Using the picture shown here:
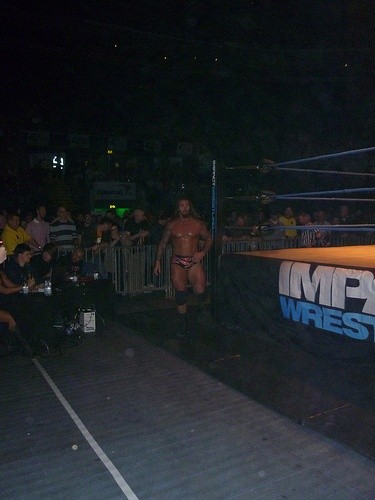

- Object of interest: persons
[154,197,213,340]
[195,198,375,290]
[0,199,173,358]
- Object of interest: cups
[22,284,29,294]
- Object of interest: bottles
[45,279,51,296]
